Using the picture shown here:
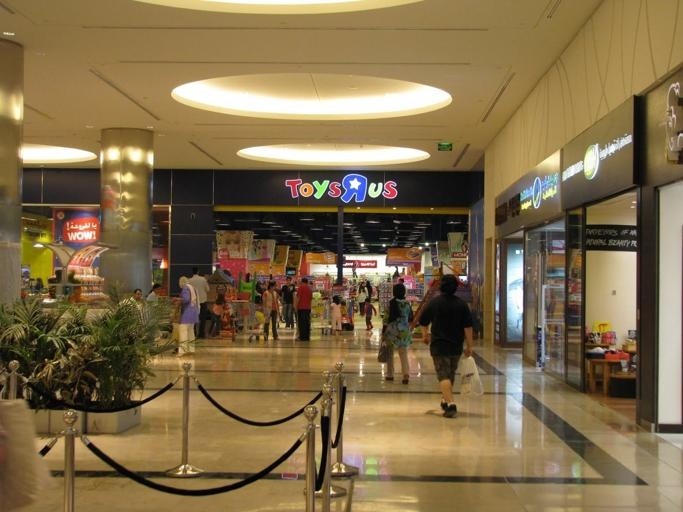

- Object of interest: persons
[330,279,372,335]
[257,277,312,340]
[364,299,377,330]
[130,272,210,356]
[379,282,413,385]
[418,274,474,418]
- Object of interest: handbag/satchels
[378,346,390,362]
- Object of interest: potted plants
[0,285,181,435]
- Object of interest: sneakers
[440,398,457,417]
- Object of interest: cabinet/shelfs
[584,336,636,395]
